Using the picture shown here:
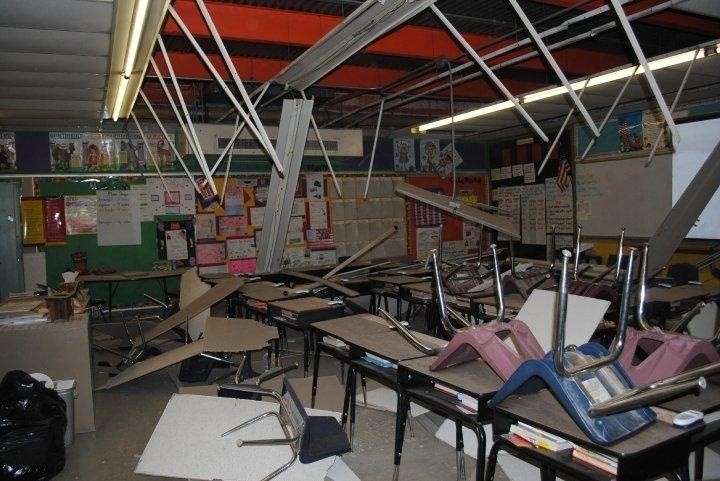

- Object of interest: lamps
[100,0,171,122]
[409,39,720,134]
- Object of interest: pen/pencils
[513,433,539,449]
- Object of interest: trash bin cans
[44,380,78,449]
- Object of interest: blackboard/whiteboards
[572,152,720,239]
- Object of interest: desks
[75,269,187,322]
[0,302,94,435]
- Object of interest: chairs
[95,221,720,481]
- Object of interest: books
[384,283,518,321]
[322,334,398,370]
[245,298,298,321]
[509,419,619,477]
[433,383,479,412]
